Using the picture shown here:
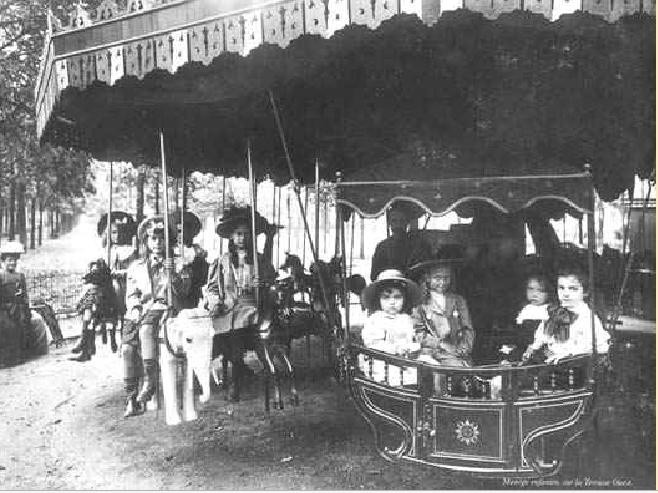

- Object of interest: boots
[73,321,93,361]
[122,375,143,417]
[136,359,157,402]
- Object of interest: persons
[69,209,284,417]
[369,206,429,275]
[0,241,34,363]
[409,248,476,370]
[498,271,557,368]
[357,269,441,393]
[490,269,612,400]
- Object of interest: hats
[407,240,468,270]
[1,242,26,253]
[98,202,270,249]
[362,270,420,310]
